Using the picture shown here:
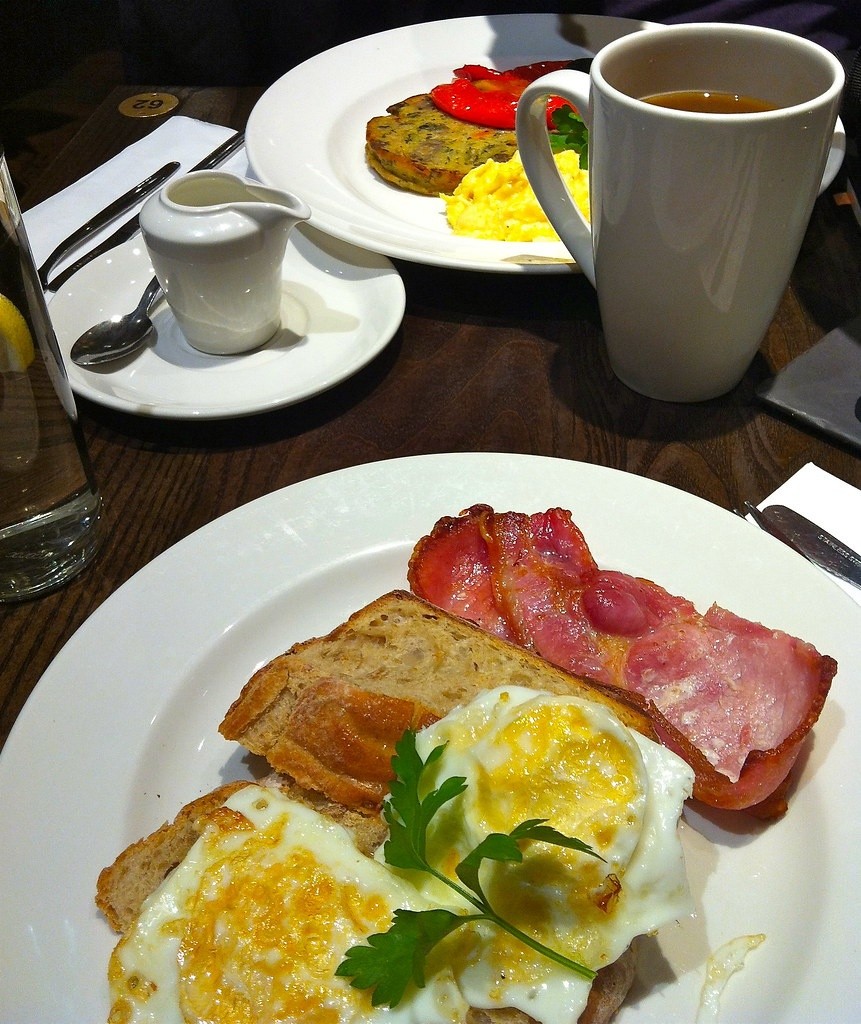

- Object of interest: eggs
[103,784,472,1024]
[374,683,697,1024]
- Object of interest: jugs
[139,169,312,356]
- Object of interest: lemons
[1,293,37,374]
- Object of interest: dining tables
[0,87,861,749]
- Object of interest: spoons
[70,274,161,365]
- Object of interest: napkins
[20,115,256,306]
[745,462,861,607]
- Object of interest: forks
[733,500,813,566]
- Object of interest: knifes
[764,505,861,590]
[47,128,245,294]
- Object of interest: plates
[22,116,406,420]
[0,451,861,1024]
[244,13,846,274]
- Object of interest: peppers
[428,62,581,129]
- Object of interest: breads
[217,587,657,844]
[96,770,640,1024]
[364,95,525,194]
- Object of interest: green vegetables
[543,102,589,171]
[333,728,610,1009]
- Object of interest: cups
[515,21,847,402]
[0,151,114,605]
